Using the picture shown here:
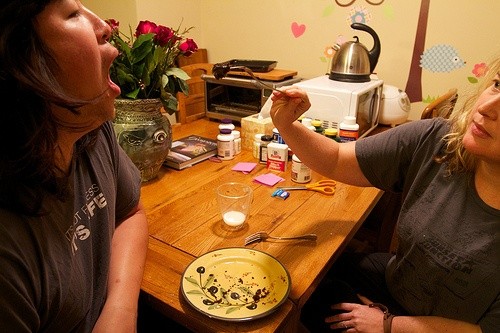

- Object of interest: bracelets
[368,303,394,333]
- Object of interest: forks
[245,230,317,246]
[230,66,287,96]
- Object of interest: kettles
[329,20,381,79]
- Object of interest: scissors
[276,180,337,195]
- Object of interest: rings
[343,321,347,328]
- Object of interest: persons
[0,0,147,333]
[269,56,500,333]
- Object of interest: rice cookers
[377,84,411,128]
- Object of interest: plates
[181,245,292,321]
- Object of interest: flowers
[104,19,198,116]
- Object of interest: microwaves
[291,74,384,138]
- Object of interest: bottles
[297,113,359,143]
[290,154,312,183]
[253,133,273,164]
[218,119,235,131]
[216,129,235,160]
[232,130,242,155]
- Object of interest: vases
[114,98,173,183]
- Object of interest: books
[162,134,218,171]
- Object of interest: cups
[272,128,285,145]
[216,182,253,232]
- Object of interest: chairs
[421,88,458,119]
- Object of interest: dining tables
[140,120,386,333]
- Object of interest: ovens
[199,74,303,122]
